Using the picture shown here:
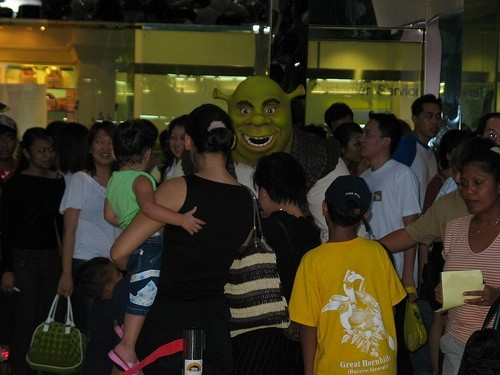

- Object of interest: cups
[182,329,203,375]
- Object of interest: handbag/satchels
[402,286,427,353]
[25,292,88,372]
[223,185,290,338]
[454,287,500,375]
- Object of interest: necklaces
[475,215,500,234]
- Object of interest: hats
[326,175,372,220]
[0,114,17,134]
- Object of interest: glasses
[486,132,500,140]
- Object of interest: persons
[0,94,500,375]
[212,77,330,193]
[104,120,206,375]
[289,175,407,375]
[110,104,258,375]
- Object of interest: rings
[481,298,483,302]
